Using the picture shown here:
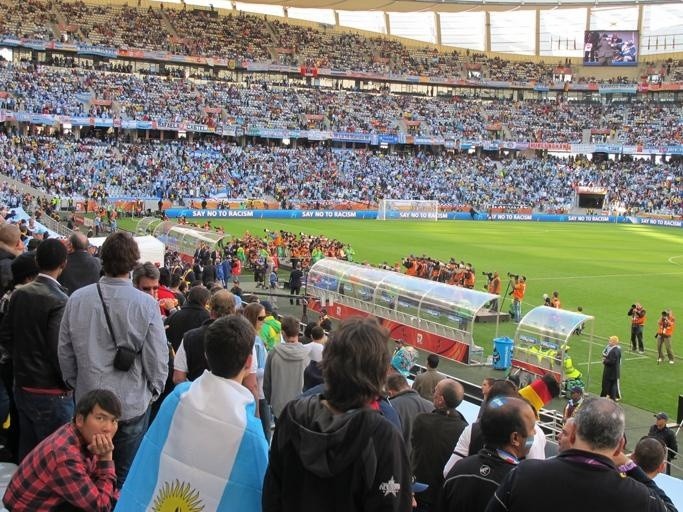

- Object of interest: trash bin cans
[492,336,514,370]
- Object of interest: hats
[395,339,403,344]
[568,386,582,394]
[654,413,667,419]
[411,472,429,493]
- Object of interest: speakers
[677,395,683,427]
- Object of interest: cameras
[632,304,636,308]
[662,311,669,317]
[507,272,518,280]
[482,272,492,281]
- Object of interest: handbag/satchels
[114,347,135,371]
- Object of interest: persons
[1,204,683,512]
[0,0,683,221]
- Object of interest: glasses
[258,317,266,321]
[143,286,158,291]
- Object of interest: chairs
[508,344,584,398]
[302,284,484,366]
[139,230,199,264]
